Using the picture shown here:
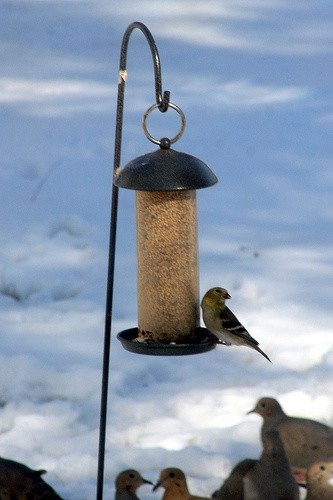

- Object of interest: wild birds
[242,430,299,500]
[304,461,333,500]
[114,470,153,500]
[200,287,272,362]
[13,470,50,500]
[210,458,260,500]
[153,468,210,500]
[244,397,333,476]
